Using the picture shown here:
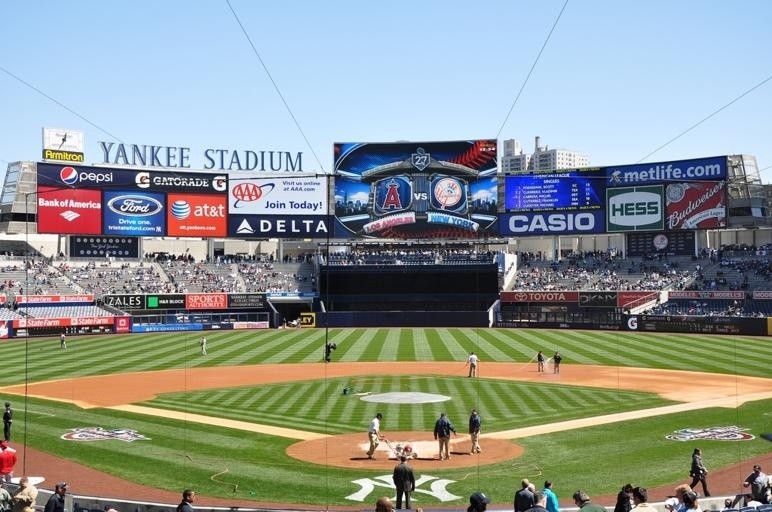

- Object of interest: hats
[572,490,589,501]
[54,481,71,490]
[751,463,762,472]
[376,412,383,418]
[683,490,701,500]
[469,491,492,503]
[1,442,8,449]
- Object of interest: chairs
[706,502,772,512]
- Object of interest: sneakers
[439,455,452,462]
[468,448,483,456]
[367,451,377,461]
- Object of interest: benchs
[1,256,132,324]
[316,249,500,270]
[133,261,315,293]
[639,250,770,317]
[506,255,641,300]
